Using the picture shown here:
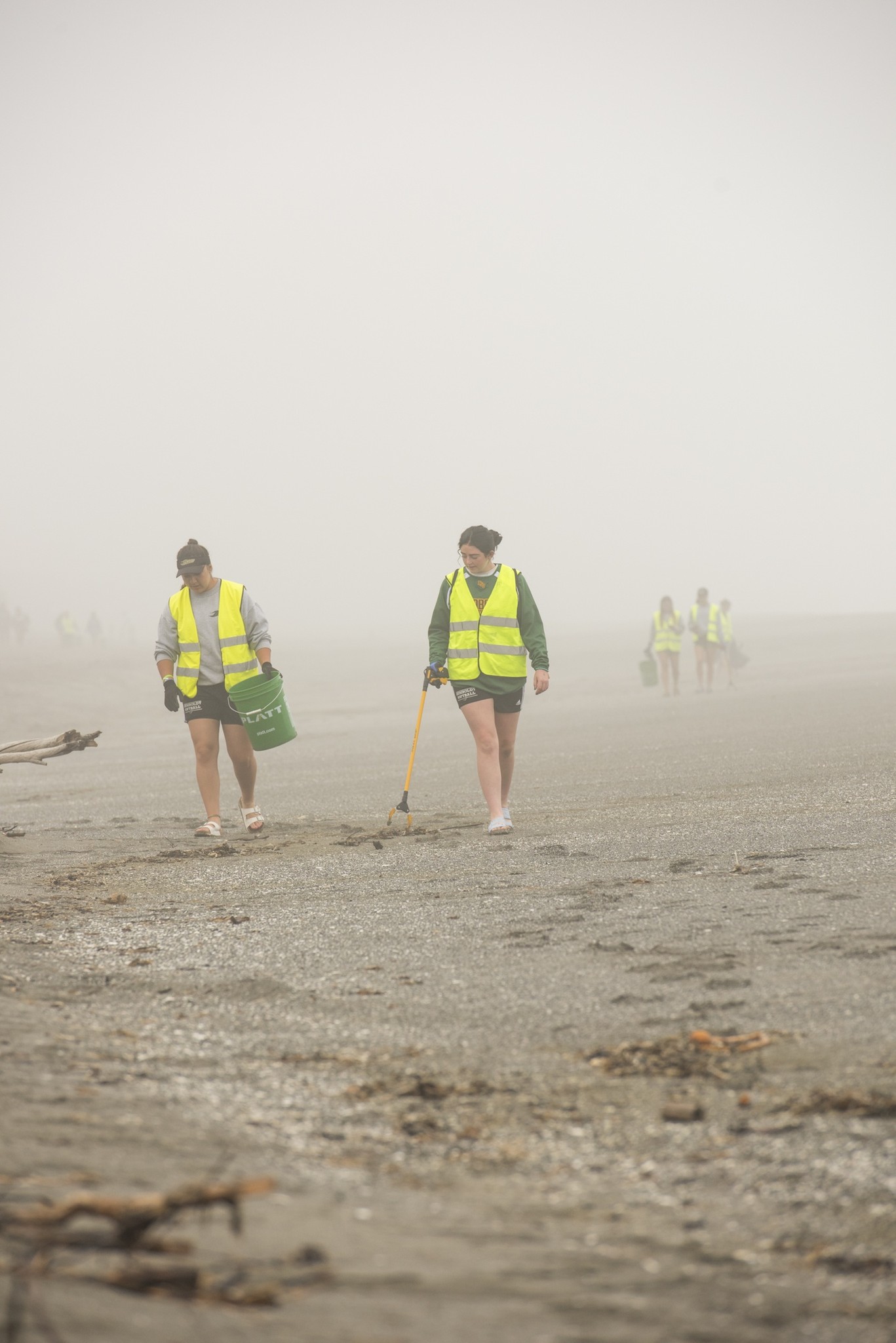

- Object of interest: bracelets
[162,674,173,681]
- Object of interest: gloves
[430,661,442,689]
[261,661,284,679]
[164,680,184,713]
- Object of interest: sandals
[238,798,264,834]
[194,822,223,836]
[488,818,508,835]
[503,808,515,834]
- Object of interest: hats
[176,544,210,579]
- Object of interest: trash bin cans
[639,661,658,686]
[228,671,297,751]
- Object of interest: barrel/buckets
[227,670,299,754]
[638,660,658,687]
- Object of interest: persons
[427,526,551,835]
[690,587,739,690]
[154,538,285,838]
[643,595,686,697]
